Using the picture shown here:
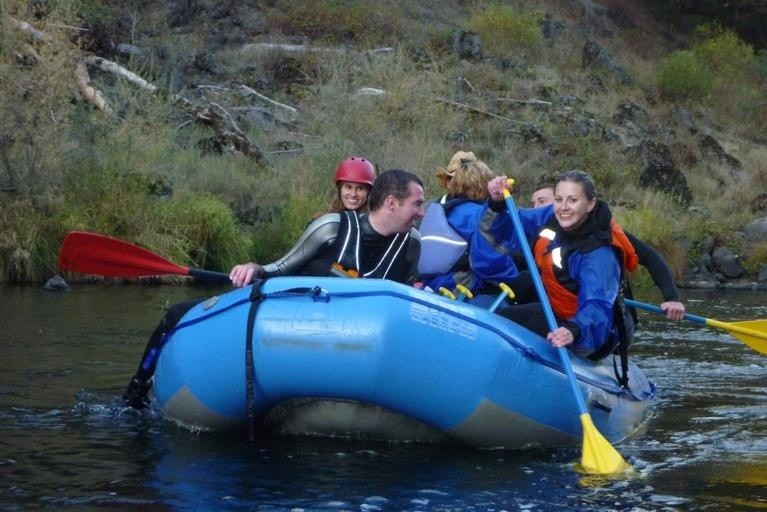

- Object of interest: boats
[152,276,657,451]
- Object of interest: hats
[437,151,477,188]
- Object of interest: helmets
[336,157,375,186]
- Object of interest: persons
[468,170,637,361]
[413,161,518,305]
[530,184,685,321]
[439,151,479,194]
[227,167,427,294]
[306,156,377,230]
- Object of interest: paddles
[623,296,766,356]
[57,232,256,283]
[498,177,632,478]
